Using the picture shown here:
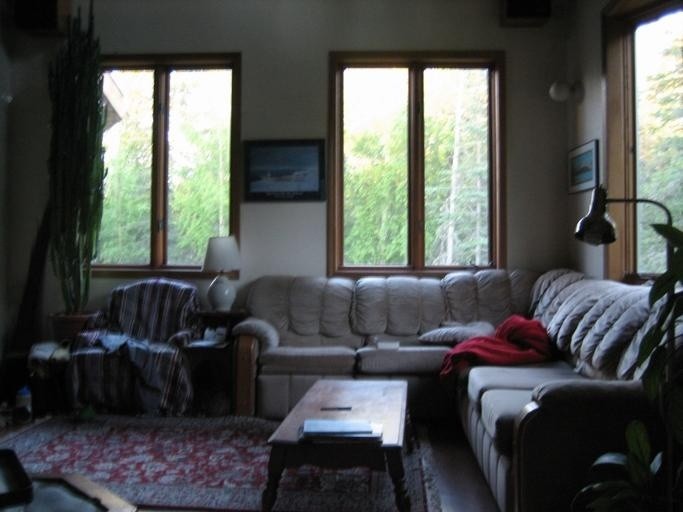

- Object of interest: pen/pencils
[319,407,351,411]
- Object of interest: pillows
[418,322,494,344]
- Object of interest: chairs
[74,278,200,418]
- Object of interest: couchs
[236,266,683,511]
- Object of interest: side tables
[186,308,243,415]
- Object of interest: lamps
[574,189,682,298]
[202,235,241,312]
[548,79,583,103]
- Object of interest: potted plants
[47,1,105,344]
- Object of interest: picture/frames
[244,138,326,201]
[567,139,600,194]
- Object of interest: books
[294,417,385,449]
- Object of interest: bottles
[14,384,33,424]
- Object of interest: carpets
[1,413,442,512]
